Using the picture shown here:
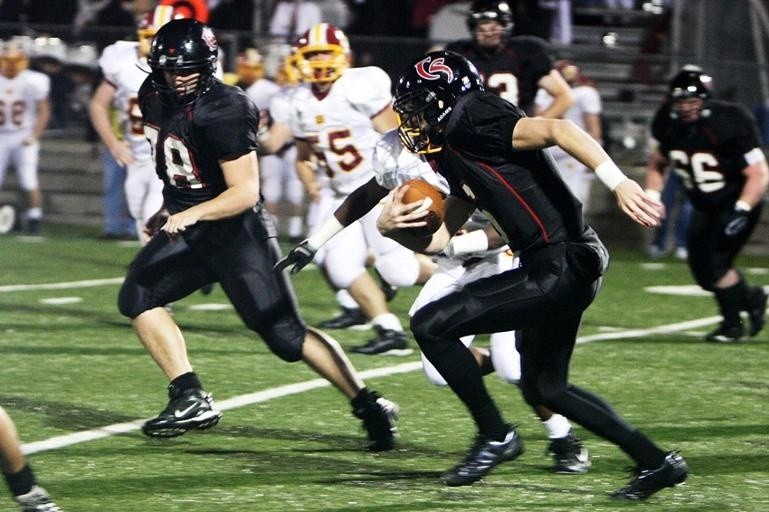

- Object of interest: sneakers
[613,451,688,503]
[318,306,368,331]
[350,328,413,355]
[546,426,591,475]
[438,425,522,486]
[707,322,744,342]
[749,285,768,338]
[15,483,62,511]
[352,392,400,450]
[143,388,220,439]
[25,220,38,235]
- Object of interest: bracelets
[645,188,661,201]
[734,201,752,212]
[594,159,626,191]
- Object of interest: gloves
[723,201,752,236]
[269,240,317,276]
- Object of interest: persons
[646,64,704,261]
[271,127,591,477]
[376,50,690,503]
[644,63,768,344]
[1,0,605,358]
[113,17,403,451]
[0,402,64,512]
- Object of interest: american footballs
[394,178,445,235]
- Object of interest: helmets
[391,50,484,154]
[669,63,714,120]
[467,1,513,59]
[285,23,350,84]
[0,45,28,76]
[234,47,265,80]
[136,7,216,108]
